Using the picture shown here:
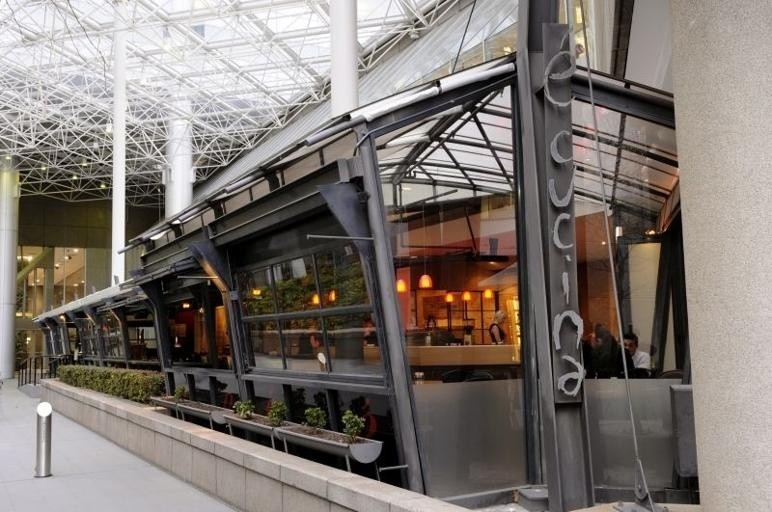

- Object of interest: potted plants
[150,385,384,464]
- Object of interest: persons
[590,327,636,379]
[488,309,508,345]
[296,319,322,360]
[363,319,379,347]
[623,332,651,371]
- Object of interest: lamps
[394,201,495,302]
